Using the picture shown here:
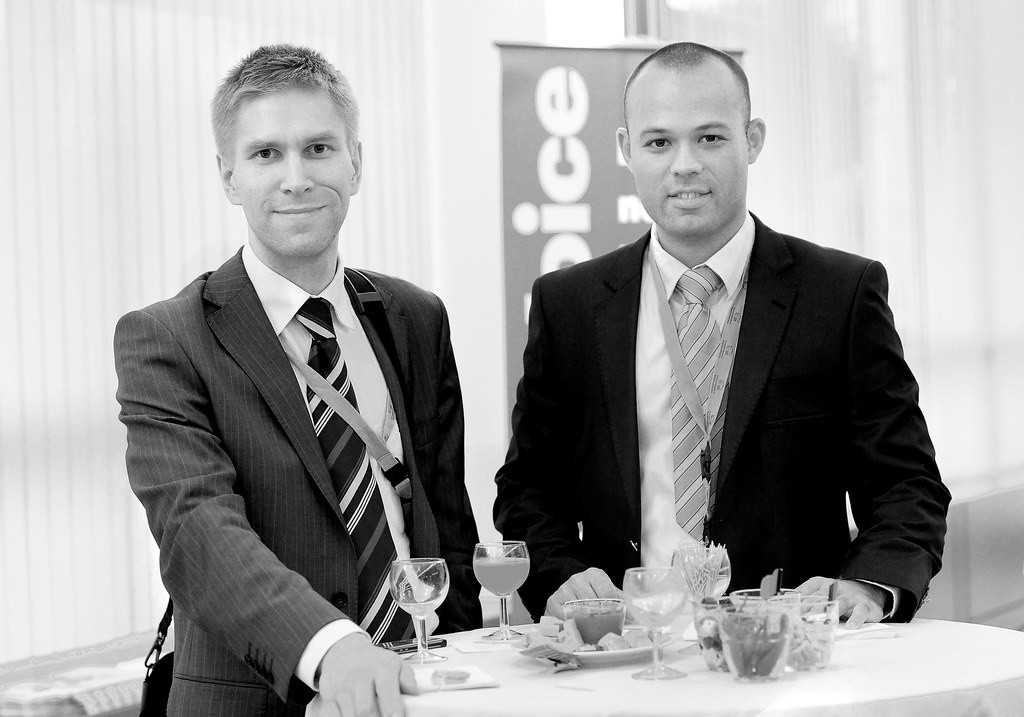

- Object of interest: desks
[305,617,1024,716]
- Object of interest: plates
[546,632,677,668]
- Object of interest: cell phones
[376,636,447,654]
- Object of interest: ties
[294,297,418,649]
[673,265,725,547]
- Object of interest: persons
[114,42,485,717]
[492,41,953,627]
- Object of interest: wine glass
[474,540,531,642]
[390,557,451,664]
[623,567,689,680]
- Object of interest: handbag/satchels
[140,650,176,717]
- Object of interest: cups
[563,598,627,645]
[671,544,840,683]
